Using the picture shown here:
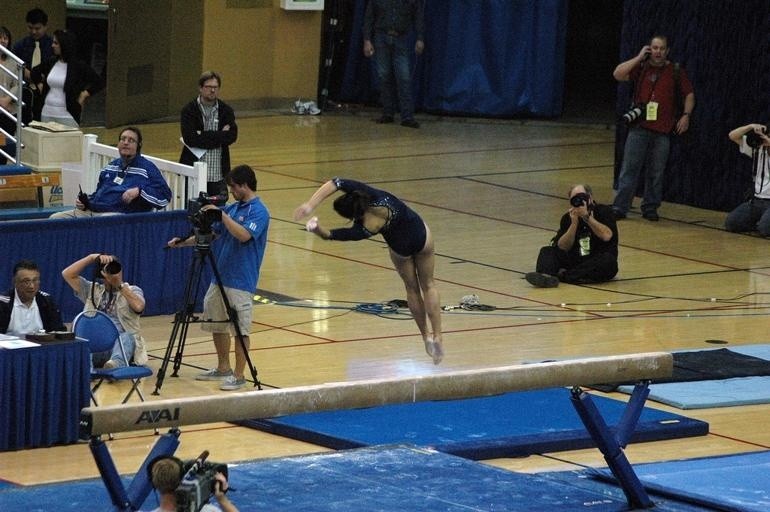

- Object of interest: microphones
[126,147,140,158]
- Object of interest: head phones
[146,454,185,489]
[138,137,142,147]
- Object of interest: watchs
[584,212,591,221]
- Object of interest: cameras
[571,192,589,208]
[623,104,646,125]
[747,131,764,146]
[94,259,122,279]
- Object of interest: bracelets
[683,111,695,119]
[117,282,129,290]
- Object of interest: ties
[29,41,41,68]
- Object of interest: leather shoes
[400,119,419,128]
[614,209,627,221]
[376,115,394,124]
[642,210,659,221]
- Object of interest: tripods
[149,248,264,394]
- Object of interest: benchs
[0,172,77,220]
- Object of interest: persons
[606,33,695,222]
[294,178,447,365]
[49,130,170,219]
[358,1,433,129]
[180,73,237,202]
[146,455,246,512]
[0,11,101,141]
[525,183,620,290]
[1,264,68,338]
[724,122,770,237]
[168,165,270,395]
[62,255,148,379]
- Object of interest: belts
[374,27,408,37]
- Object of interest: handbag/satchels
[675,62,705,137]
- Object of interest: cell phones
[645,52,651,59]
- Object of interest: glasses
[120,136,138,145]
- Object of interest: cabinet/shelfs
[20,125,83,208]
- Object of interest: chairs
[72,309,159,440]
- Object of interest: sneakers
[103,359,117,382]
[220,375,247,391]
[558,268,568,282]
[289,101,307,115]
[526,272,559,287]
[305,101,321,116]
[196,368,233,381]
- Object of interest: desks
[0,332,90,451]
